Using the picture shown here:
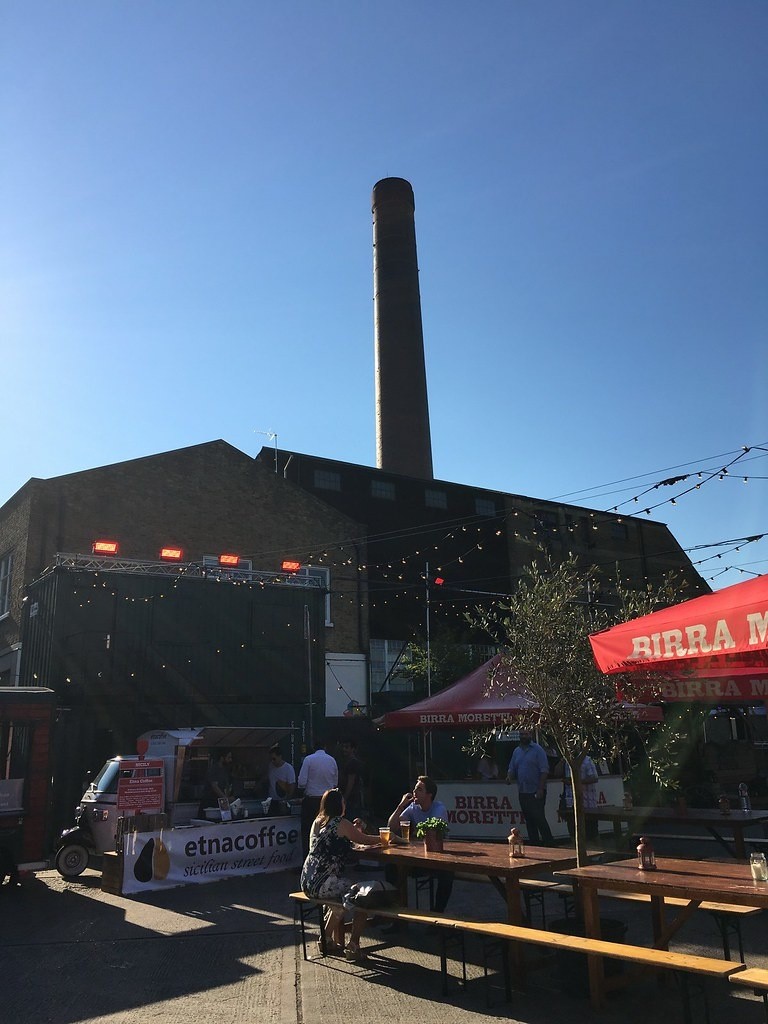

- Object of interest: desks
[359,835,607,992]
[552,855,768,1018]
[556,802,768,866]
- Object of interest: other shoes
[378,918,408,933]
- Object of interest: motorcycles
[55,727,307,879]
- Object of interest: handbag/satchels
[558,791,566,811]
[341,879,401,910]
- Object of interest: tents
[385,651,664,779]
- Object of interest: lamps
[281,560,302,575]
[93,539,119,555]
[159,546,184,560]
[219,554,241,568]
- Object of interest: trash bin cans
[547,916,629,985]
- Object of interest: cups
[379,827,390,846]
[400,821,410,840]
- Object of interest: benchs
[289,834,768,1015]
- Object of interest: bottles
[739,780,751,813]
[750,852,767,881]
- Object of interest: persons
[267,747,296,814]
[477,755,498,780]
[298,738,339,859]
[385,775,454,913]
[336,745,361,818]
[506,727,554,847]
[301,789,384,958]
[198,748,232,818]
[555,738,599,847]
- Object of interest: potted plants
[415,818,450,853]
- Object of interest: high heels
[318,936,345,954]
[344,941,370,960]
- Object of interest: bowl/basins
[204,809,221,818]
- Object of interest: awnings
[588,573,768,673]
[615,668,768,704]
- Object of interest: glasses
[271,758,276,761]
[335,787,341,794]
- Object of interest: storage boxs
[100,850,122,895]
